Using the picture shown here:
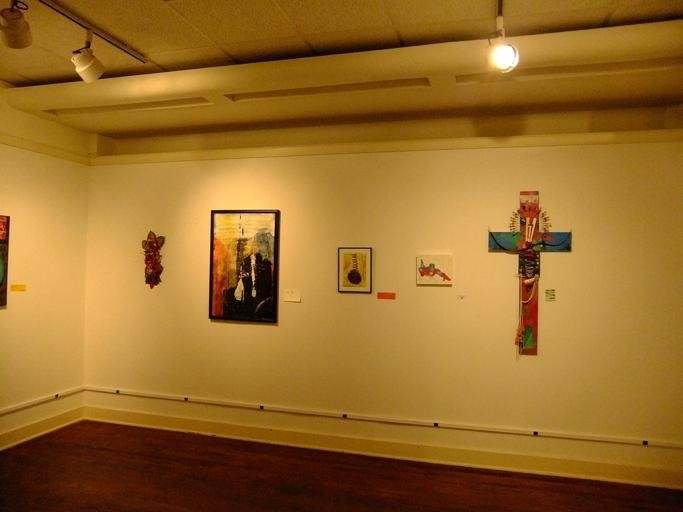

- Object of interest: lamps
[0,0,107,86]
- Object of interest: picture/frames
[206,208,280,326]
[336,247,372,295]
